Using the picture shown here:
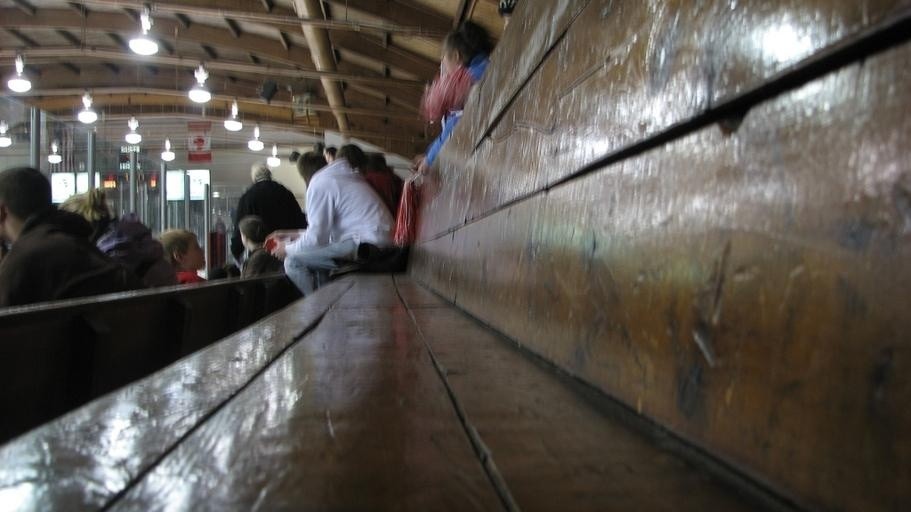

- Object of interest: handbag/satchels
[354,243,406,273]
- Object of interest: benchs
[0,0,909,510]
[0,275,299,444]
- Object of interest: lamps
[77,92,99,126]
[269,143,279,168]
[186,62,213,106]
[4,55,35,92]
[159,136,178,162]
[47,143,62,164]
[123,115,143,146]
[125,3,162,58]
[2,120,12,150]
[245,122,265,151]
[222,98,245,132]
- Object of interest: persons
[416,19,488,172]
[0,168,205,309]
[238,214,279,287]
[230,161,308,265]
[267,147,409,296]
